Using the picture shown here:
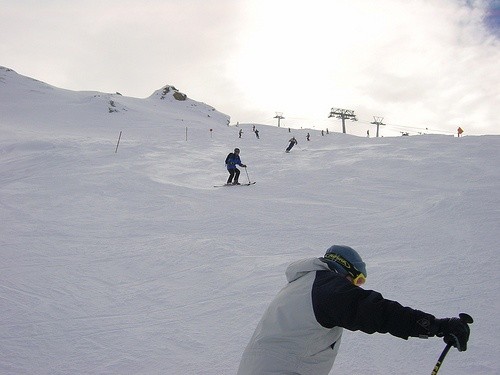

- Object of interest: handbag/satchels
[226,153,234,164]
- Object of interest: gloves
[436,318,470,352]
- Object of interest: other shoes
[227,182,232,184]
[234,181,238,183]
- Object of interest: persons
[252,125,255,131]
[305,133,311,141]
[457,127,463,137]
[236,243,471,375]
[239,129,244,138]
[326,128,329,134]
[288,128,291,132]
[367,129,369,137]
[320,130,325,136]
[284,136,298,153]
[225,147,249,185]
[255,129,260,139]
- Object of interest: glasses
[324,253,366,286]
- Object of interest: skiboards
[214,182,256,187]
[284,149,291,153]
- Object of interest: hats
[235,148,240,152]
[324,244,367,280]
